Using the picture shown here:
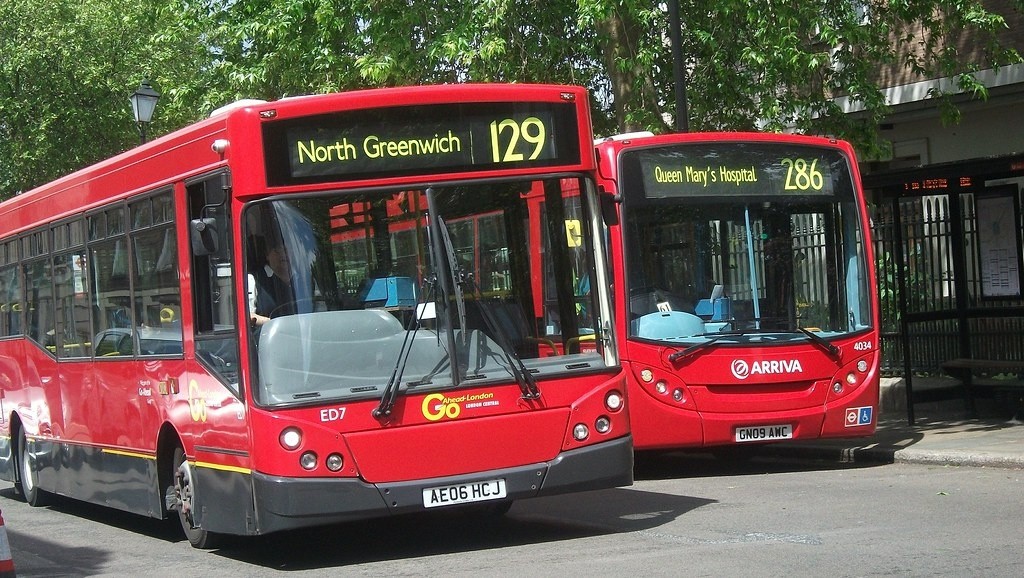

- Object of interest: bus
[0,82,634,551]
[326,130,881,471]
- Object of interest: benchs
[942,358,1024,418]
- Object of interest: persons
[247,234,327,328]
[577,244,639,325]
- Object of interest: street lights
[129,75,161,146]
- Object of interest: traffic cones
[0,509,17,578]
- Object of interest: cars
[92,324,240,403]
